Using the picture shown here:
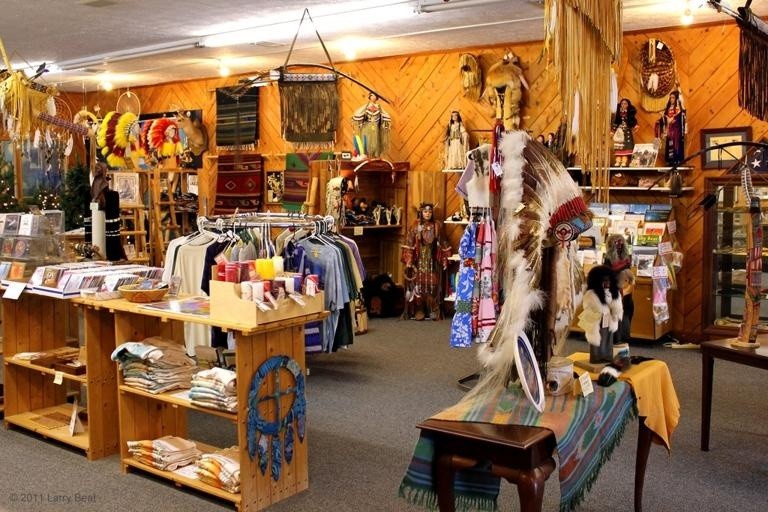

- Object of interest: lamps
[55,37,205,71]
[411,0,495,14]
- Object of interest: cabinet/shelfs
[103,294,331,512]
[701,175,768,337]
[442,167,695,339]
[0,285,120,460]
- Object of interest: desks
[416,352,666,512]
[700,334,767,452]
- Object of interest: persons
[610,99,640,167]
[351,92,392,160]
[444,111,469,168]
[603,234,634,344]
[545,133,554,148]
[655,91,685,167]
[536,135,546,146]
[577,267,623,364]
[401,204,452,321]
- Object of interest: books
[576,203,674,277]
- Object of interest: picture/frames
[700,126,752,170]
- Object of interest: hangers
[186,210,342,258]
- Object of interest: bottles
[240,272,319,302]
[92,208,107,260]
[84,217,92,242]
[544,356,574,397]
[211,256,284,283]
[613,342,631,370]
